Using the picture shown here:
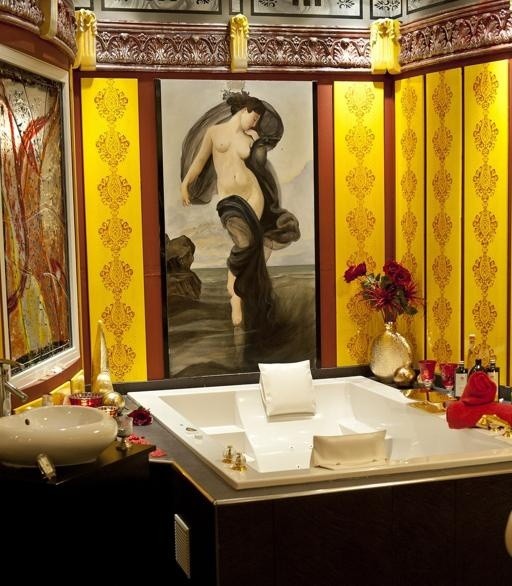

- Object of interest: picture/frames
[0,42,82,391]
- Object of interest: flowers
[343,258,425,322]
[126,406,152,426]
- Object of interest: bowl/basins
[68,391,118,417]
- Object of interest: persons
[181,92,299,365]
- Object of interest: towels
[446,400,512,429]
[461,371,497,405]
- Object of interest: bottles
[455,358,500,402]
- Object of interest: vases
[367,323,414,383]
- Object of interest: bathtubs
[127,376,512,490]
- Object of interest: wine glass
[418,360,458,403]
[116,417,135,451]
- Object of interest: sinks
[1,404,118,469]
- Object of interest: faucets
[0,359,27,417]
